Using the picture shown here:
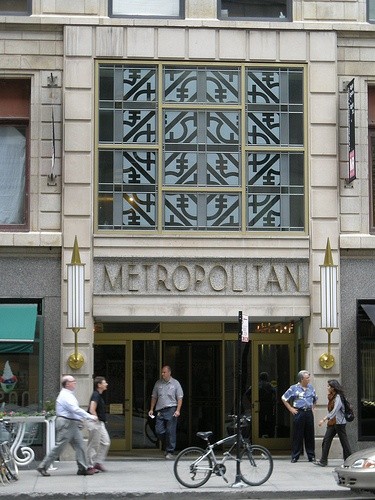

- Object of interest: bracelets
[323,419,325,421]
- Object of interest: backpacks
[340,399,354,422]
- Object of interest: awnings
[360,304,375,326]
[0,303,38,353]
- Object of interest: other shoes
[291,457,297,462]
[94,463,105,473]
[316,462,324,466]
[310,458,316,461]
[165,452,174,459]
[37,467,50,476]
[78,467,94,474]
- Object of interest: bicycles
[173,414,273,488]
[0,419,20,482]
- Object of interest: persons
[86,376,111,472]
[245,372,276,437]
[148,365,184,459]
[312,380,352,467]
[281,370,317,463]
[37,375,98,477]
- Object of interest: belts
[299,408,312,411]
